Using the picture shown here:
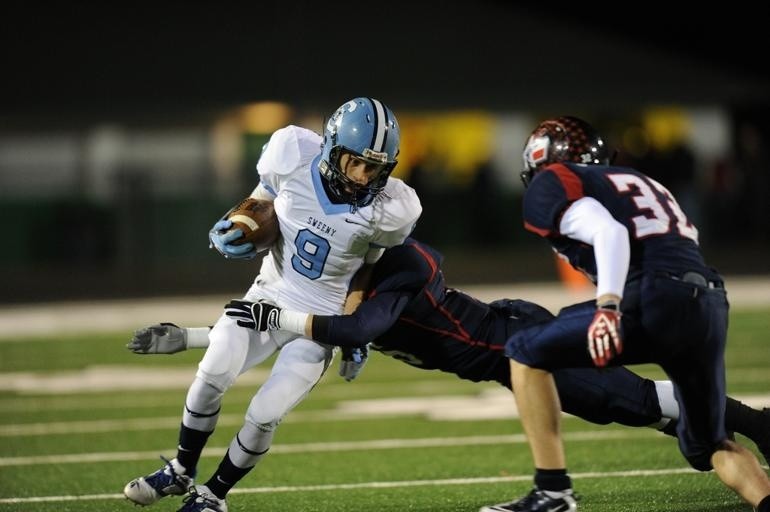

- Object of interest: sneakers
[123,458,195,507]
[478,486,578,512]
[176,486,227,512]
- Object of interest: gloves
[586,308,624,367]
[339,344,369,382]
[223,298,287,331]
[208,219,257,262]
[125,322,187,355]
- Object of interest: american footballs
[226,199,280,253]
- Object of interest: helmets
[322,96,401,165]
[520,116,611,179]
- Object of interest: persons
[122,99,423,512]
[125,233,769,468]
[475,116,770,512]
